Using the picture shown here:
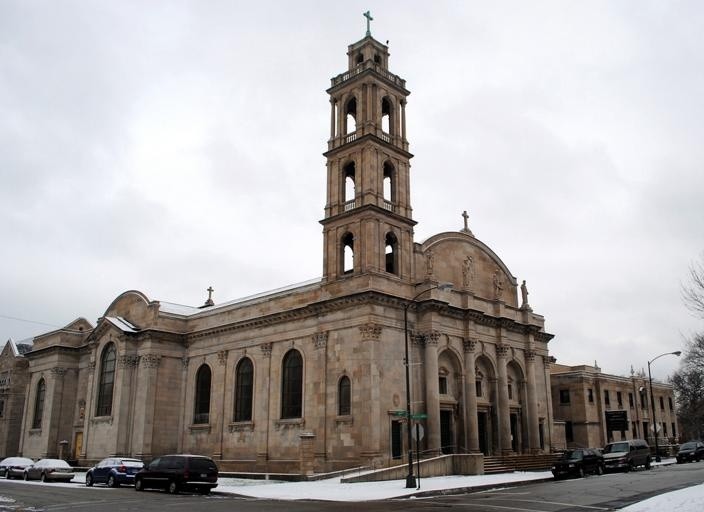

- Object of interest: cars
[552,448,605,480]
[676,442,704,464]
[86,458,145,486]
[0,457,34,480]
[23,459,74,481]
[134,456,218,494]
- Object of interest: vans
[602,439,651,473]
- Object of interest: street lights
[404,283,454,488]
[648,351,681,462]
